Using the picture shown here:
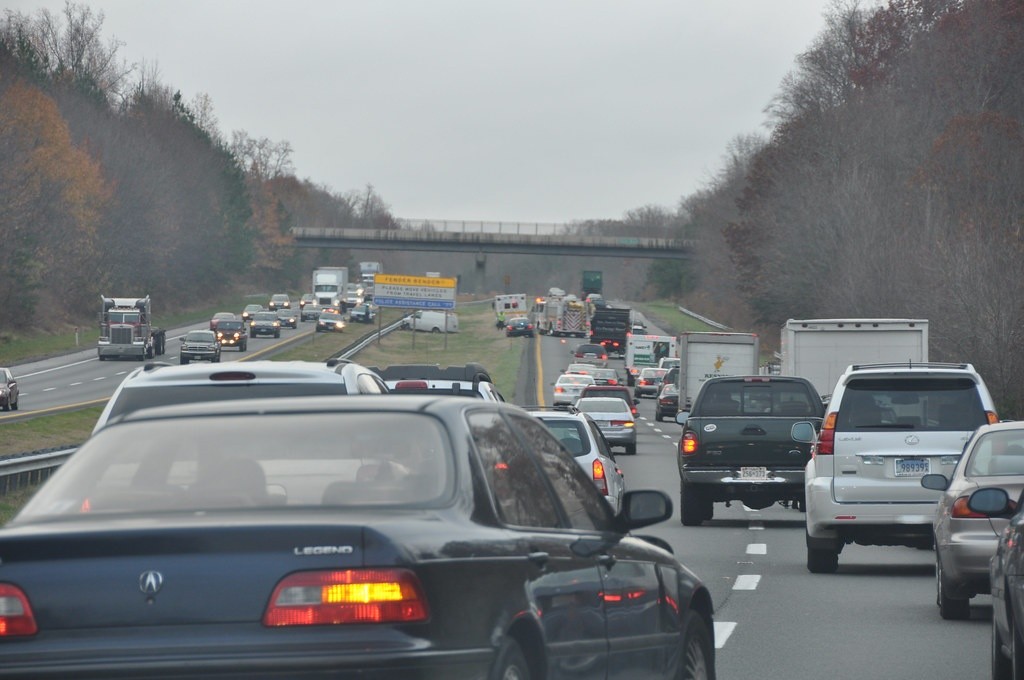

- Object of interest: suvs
[214,320,248,352]
[85,356,409,509]
[178,329,222,364]
[789,360,1000,573]
[674,374,829,526]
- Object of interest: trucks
[401,309,459,334]
[780,319,929,429]
[677,331,760,420]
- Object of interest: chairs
[770,400,809,416]
[701,399,741,417]
[987,453,1024,476]
[560,437,582,454]
[848,394,883,427]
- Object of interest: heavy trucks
[97,292,166,360]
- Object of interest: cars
[965,486,1024,680]
[917,419,1024,621]
[0,393,718,680]
[242,261,384,339]
[0,366,21,413]
[492,268,683,455]
[515,403,627,520]
[210,312,236,330]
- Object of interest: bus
[361,363,507,407]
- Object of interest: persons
[497,311,505,331]
